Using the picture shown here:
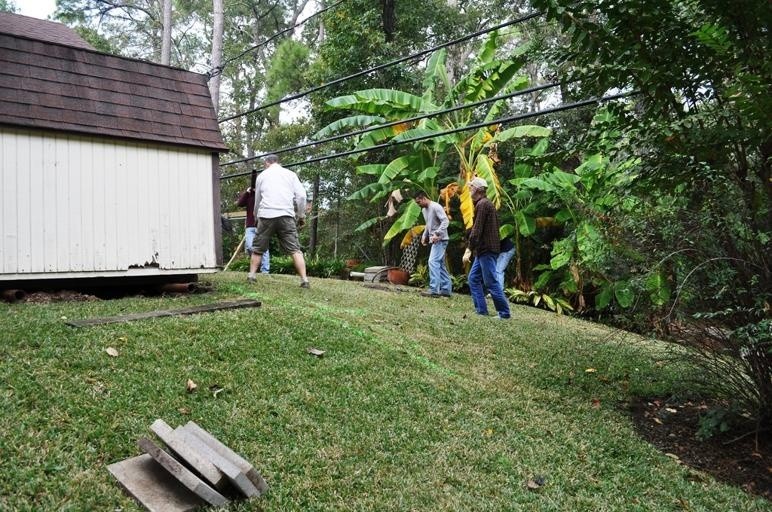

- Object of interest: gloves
[462,248,473,263]
[296,217,305,226]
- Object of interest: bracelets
[465,251,471,253]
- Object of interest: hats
[467,178,489,190]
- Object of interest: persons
[461,176,513,322]
[235,187,271,275]
[246,154,311,290]
[413,190,453,299]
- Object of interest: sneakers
[440,293,451,297]
[246,276,257,282]
[300,283,309,288]
[491,315,500,320]
[422,292,438,298]
